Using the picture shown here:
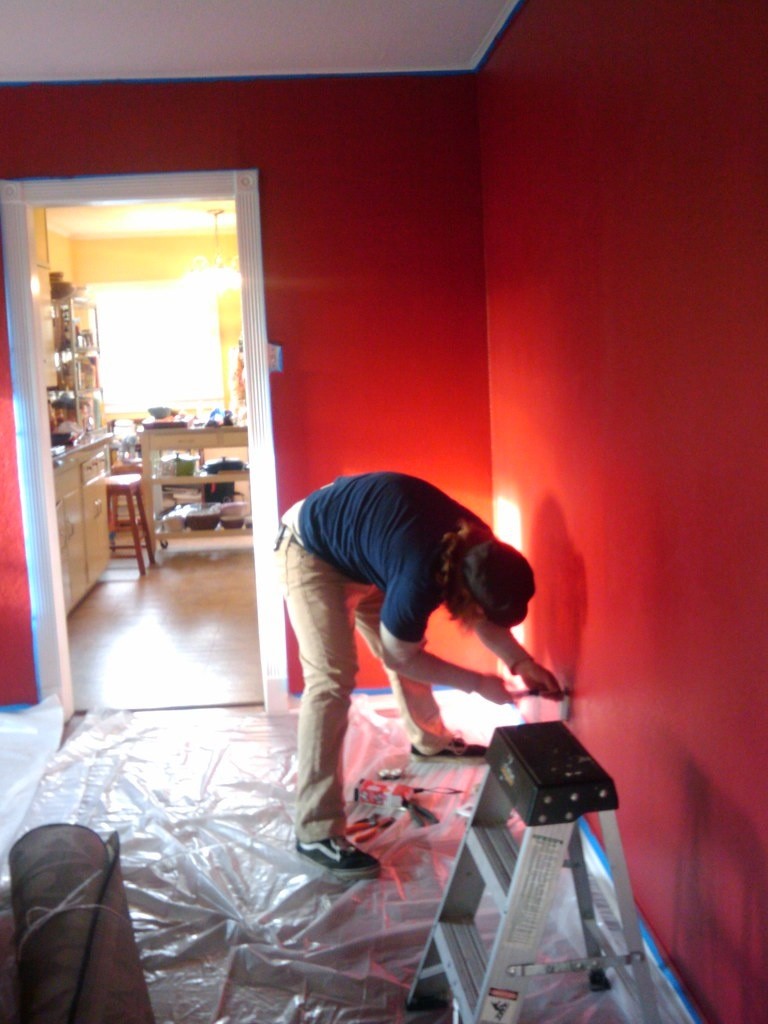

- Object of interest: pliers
[403,794,440,828]
[346,816,395,844]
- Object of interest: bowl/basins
[220,518,244,529]
[186,514,220,530]
[148,408,170,419]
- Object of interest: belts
[282,526,300,546]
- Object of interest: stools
[105,474,155,576]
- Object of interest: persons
[273,471,563,878]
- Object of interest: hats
[464,540,535,628]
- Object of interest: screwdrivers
[510,688,565,699]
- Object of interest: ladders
[403,720,659,1024]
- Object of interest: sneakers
[411,730,488,764]
[296,835,380,878]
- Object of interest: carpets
[9,824,156,1024]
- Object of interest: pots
[203,456,244,474]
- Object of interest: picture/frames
[33,207,52,269]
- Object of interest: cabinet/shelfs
[140,424,253,548]
[53,298,102,438]
[51,432,114,616]
